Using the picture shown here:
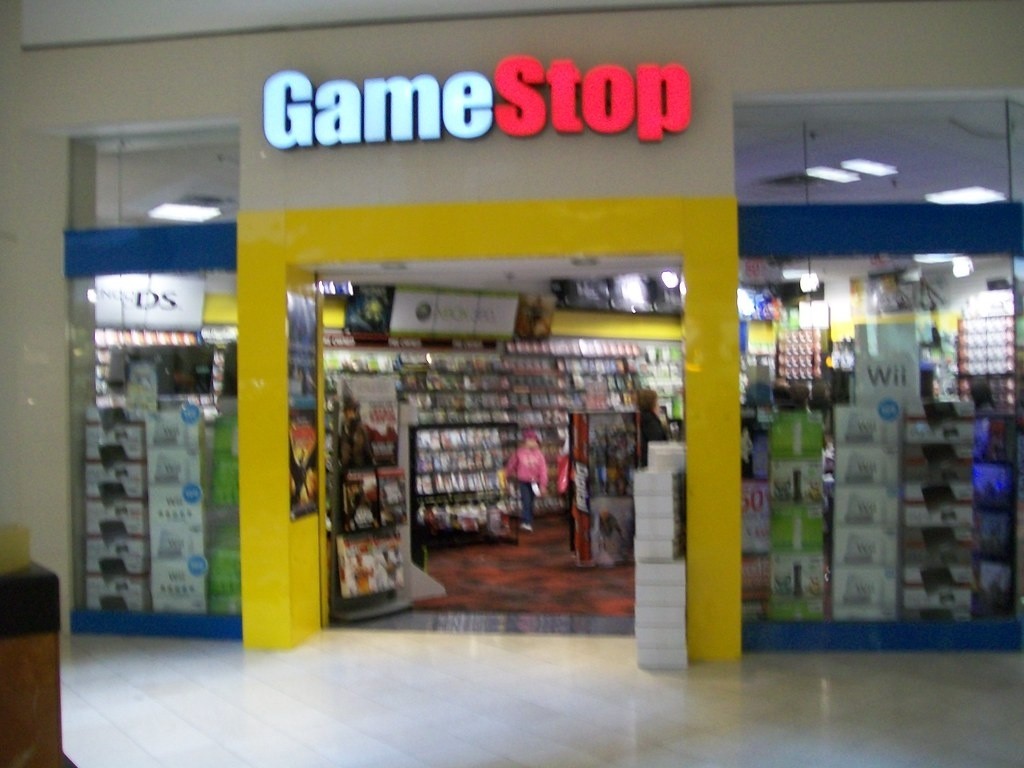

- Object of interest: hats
[523,430,537,442]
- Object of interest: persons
[507,431,548,532]
[343,539,402,598]
[638,390,668,443]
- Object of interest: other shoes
[519,524,533,534]
